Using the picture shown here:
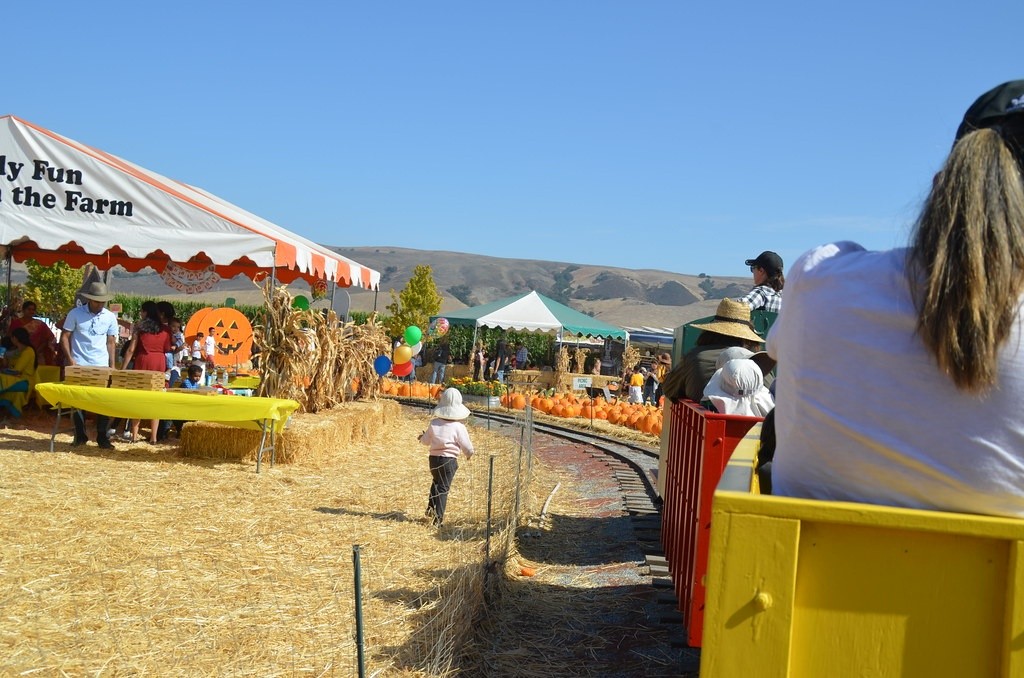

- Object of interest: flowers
[442,377,506,397]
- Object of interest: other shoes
[425,507,434,517]
[176,431,181,439]
[70,436,86,447]
[122,430,131,440]
[433,521,443,527]
[107,428,117,435]
[99,442,115,450]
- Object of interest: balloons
[373,318,450,376]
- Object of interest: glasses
[751,267,760,273]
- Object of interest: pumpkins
[498,389,663,436]
[382,380,443,399]
[223,363,264,377]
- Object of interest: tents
[0,115,381,339]
[430,290,630,354]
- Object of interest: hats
[658,352,672,364]
[434,388,470,420]
[640,367,647,373]
[745,251,783,276]
[953,79,1024,171]
[689,298,767,342]
[715,345,775,376]
[81,282,115,302]
[702,358,775,417]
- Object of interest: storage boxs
[64,365,166,392]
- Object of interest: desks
[512,369,540,391]
[36,381,300,475]
[175,373,261,389]
[37,365,60,405]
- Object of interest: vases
[462,393,500,407]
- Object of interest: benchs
[509,382,542,385]
[698,422,1024,678]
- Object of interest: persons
[622,352,672,408]
[664,251,786,417]
[756,78,1024,519]
[474,339,553,386]
[417,388,474,528]
[393,333,450,384]
[0,282,224,450]
